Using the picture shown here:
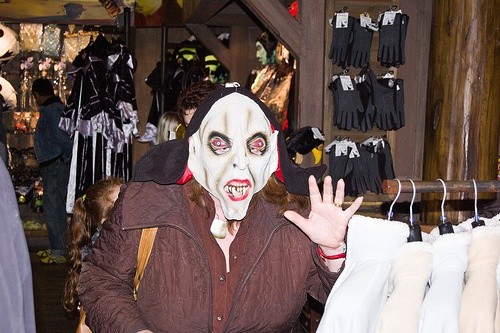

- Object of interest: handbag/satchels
[76,227,158,333]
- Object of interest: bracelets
[318,243,346,259]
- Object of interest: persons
[32,77,75,263]
[62,178,128,333]
[247,31,276,101]
[77,85,364,333]
[176,80,216,127]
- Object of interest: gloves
[325,5,410,197]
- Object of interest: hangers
[388,178,485,242]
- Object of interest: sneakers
[37,249,52,257]
[40,254,69,265]
